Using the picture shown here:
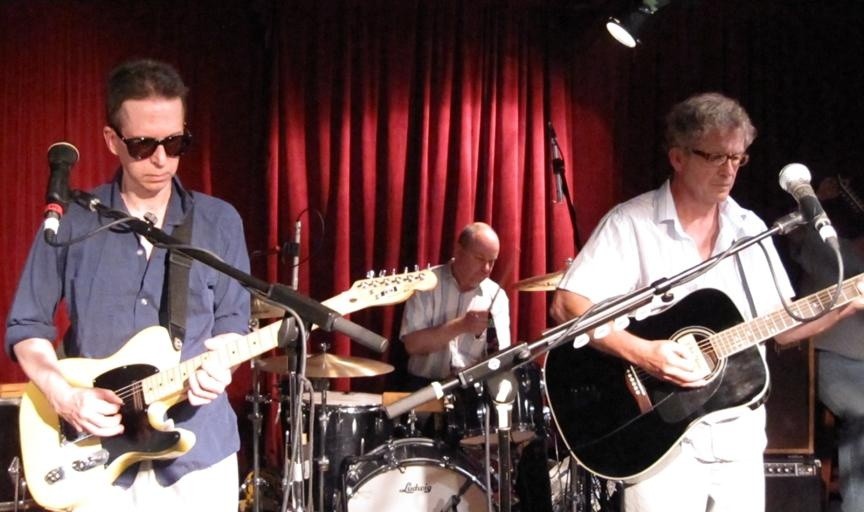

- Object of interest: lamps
[605,0,690,48]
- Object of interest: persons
[3,57,256,511]
[548,91,864,512]
[398,220,515,438]
[788,175,863,512]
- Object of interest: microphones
[42,139,82,241]
[547,142,562,200]
[778,162,841,250]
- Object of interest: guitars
[19,263,438,511]
[543,272,859,486]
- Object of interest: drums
[444,358,551,449]
[331,438,501,512]
[286,390,393,511]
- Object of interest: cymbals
[251,298,288,321]
[254,351,395,380]
[511,272,566,292]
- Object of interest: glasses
[687,147,749,168]
[110,120,193,161]
